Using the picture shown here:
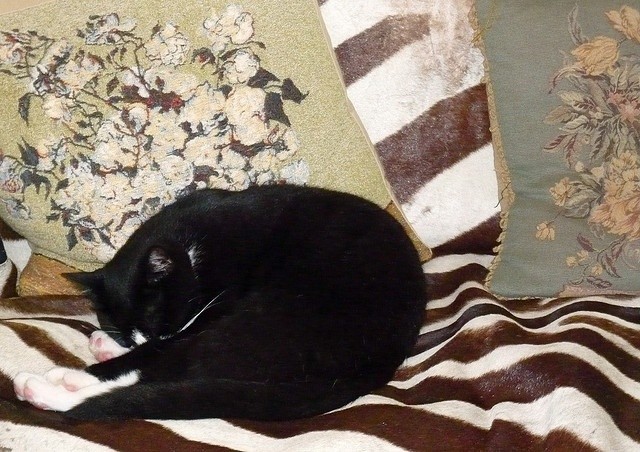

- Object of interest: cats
[12,180,429,429]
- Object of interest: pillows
[0,1,433,274]
[312,1,504,256]
[467,1,640,302]
